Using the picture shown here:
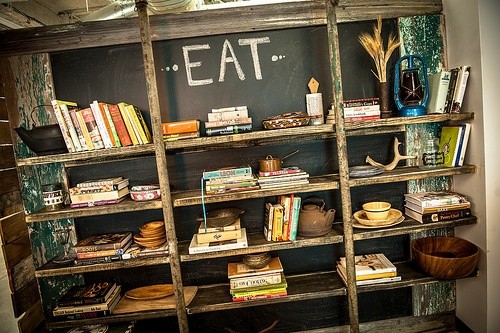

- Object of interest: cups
[40,183,65,211]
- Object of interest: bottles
[418,137,446,170]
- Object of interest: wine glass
[52,229,74,260]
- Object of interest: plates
[134,221,167,249]
[352,216,406,229]
[124,284,174,300]
[354,209,402,226]
[348,166,385,178]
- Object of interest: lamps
[393,54,430,115]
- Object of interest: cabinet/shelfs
[0,0,481,333]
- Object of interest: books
[325,97,380,124]
[336,253,401,288]
[204,117,253,137]
[436,123,471,169]
[188,216,248,255]
[51,99,153,153]
[263,194,302,242]
[160,119,201,143]
[425,65,471,114]
[68,176,131,208]
[228,257,288,302]
[202,164,310,195]
[52,281,121,322]
[403,190,472,224]
[72,231,179,266]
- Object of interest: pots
[258,149,300,171]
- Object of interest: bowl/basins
[128,186,162,201]
[194,207,245,227]
[362,200,391,220]
[411,236,480,279]
[242,252,271,269]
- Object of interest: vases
[380,82,392,118]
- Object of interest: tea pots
[13,105,69,156]
[297,196,335,238]
